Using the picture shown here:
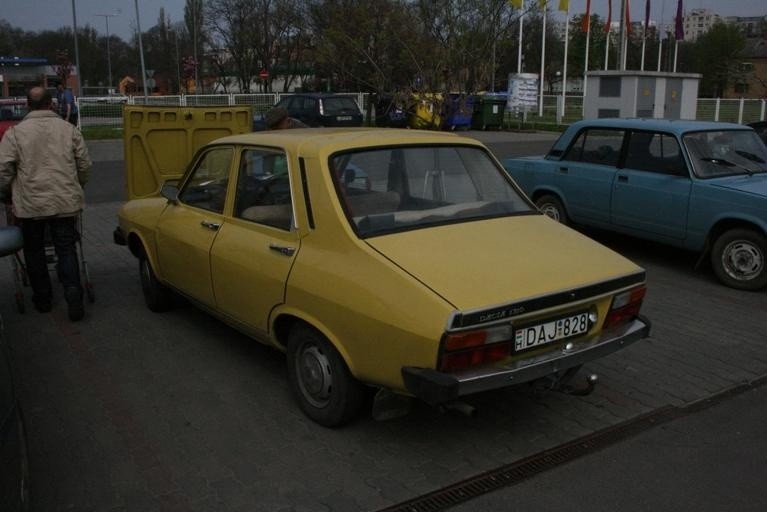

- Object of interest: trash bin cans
[374,92,508,131]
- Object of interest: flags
[509,0,522,10]
[538,1,545,13]
[644,1,650,38]
[580,1,590,33]
[674,1,684,41]
[558,1,568,13]
[602,0,611,33]
[624,1,631,38]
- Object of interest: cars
[110,122,656,434]
[1,97,57,143]
[716,121,766,165]
[98,93,130,105]
[498,116,766,294]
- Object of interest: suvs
[261,91,366,131]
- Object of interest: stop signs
[260,71,271,81]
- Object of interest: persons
[262,107,314,129]
[0,87,94,321]
[53,81,78,127]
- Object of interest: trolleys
[5,192,99,318]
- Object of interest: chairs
[240,189,496,231]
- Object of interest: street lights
[168,29,184,106]
[95,12,123,98]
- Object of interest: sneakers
[65,286,86,323]
[32,291,53,314]
